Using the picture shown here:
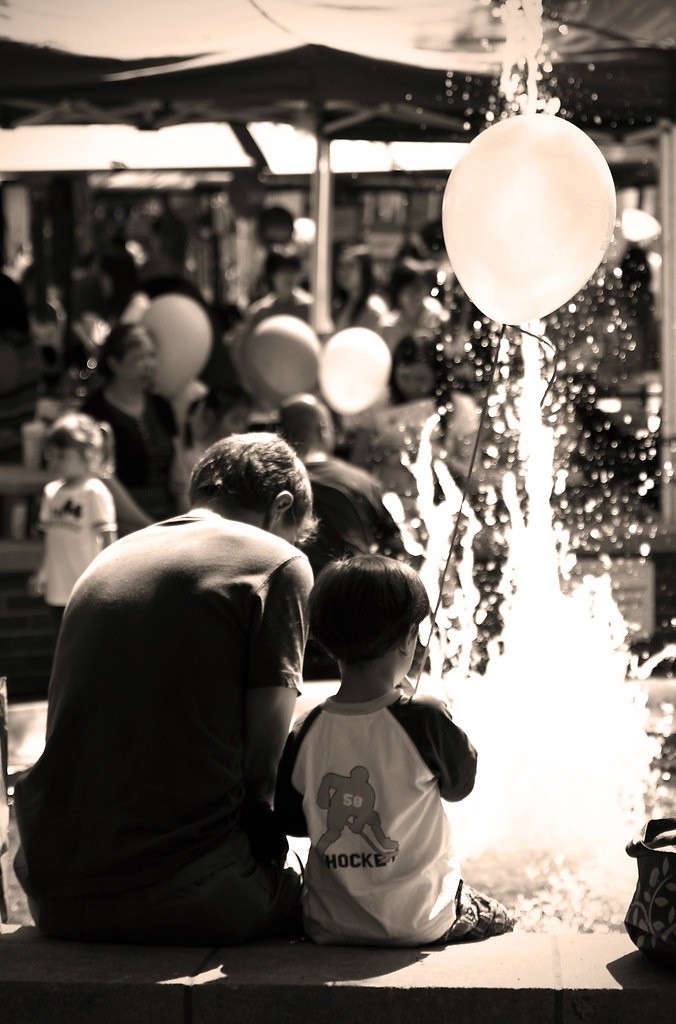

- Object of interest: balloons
[136,293,213,398]
[317,327,396,415]
[443,113,618,323]
[245,313,321,394]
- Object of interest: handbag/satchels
[624,818,676,964]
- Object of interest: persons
[0,195,660,702]
[12,431,321,947]
[272,554,515,948]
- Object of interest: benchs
[0,923,676,1024]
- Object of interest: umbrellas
[59,28,510,335]
